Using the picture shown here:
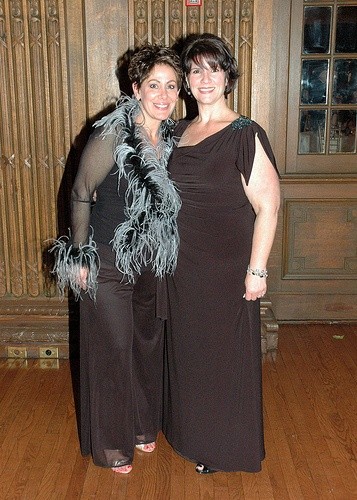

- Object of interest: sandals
[134,435,156,453]
[102,449,133,474]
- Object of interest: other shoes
[196,463,218,474]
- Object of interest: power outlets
[7,346,27,359]
[39,348,58,359]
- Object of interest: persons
[161,34,281,474]
[44,46,184,474]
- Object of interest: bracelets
[246,267,269,277]
